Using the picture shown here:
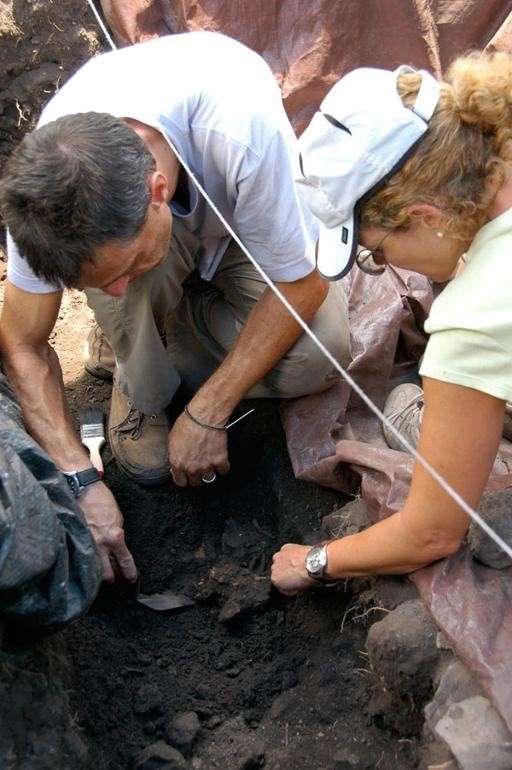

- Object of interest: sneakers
[382,382,420,460]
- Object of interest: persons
[268,46,512,598]
[1,28,354,588]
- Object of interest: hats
[291,64,440,280]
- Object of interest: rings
[200,472,217,485]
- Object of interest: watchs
[303,539,345,588]
[62,467,106,500]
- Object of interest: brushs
[79,406,107,477]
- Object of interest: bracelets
[183,403,227,434]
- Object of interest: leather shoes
[85,325,119,379]
[109,366,175,487]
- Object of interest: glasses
[356,223,396,276]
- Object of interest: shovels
[112,552,196,613]
